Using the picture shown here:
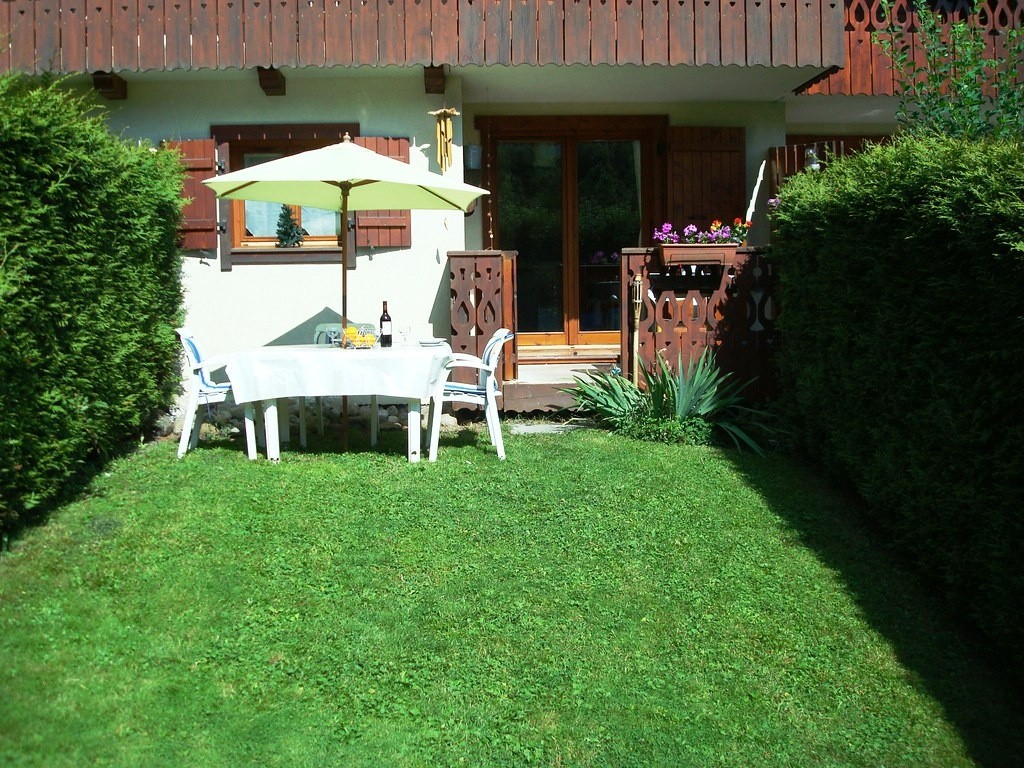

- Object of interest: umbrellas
[201,132,491,453]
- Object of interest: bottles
[380,302,392,347]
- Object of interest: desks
[225,333,452,463]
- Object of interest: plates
[420,344,443,346]
[418,338,448,344]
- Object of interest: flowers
[590,250,620,265]
[656,213,751,246]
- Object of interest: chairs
[177,339,265,460]
[426,329,514,464]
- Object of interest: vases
[664,244,743,265]
